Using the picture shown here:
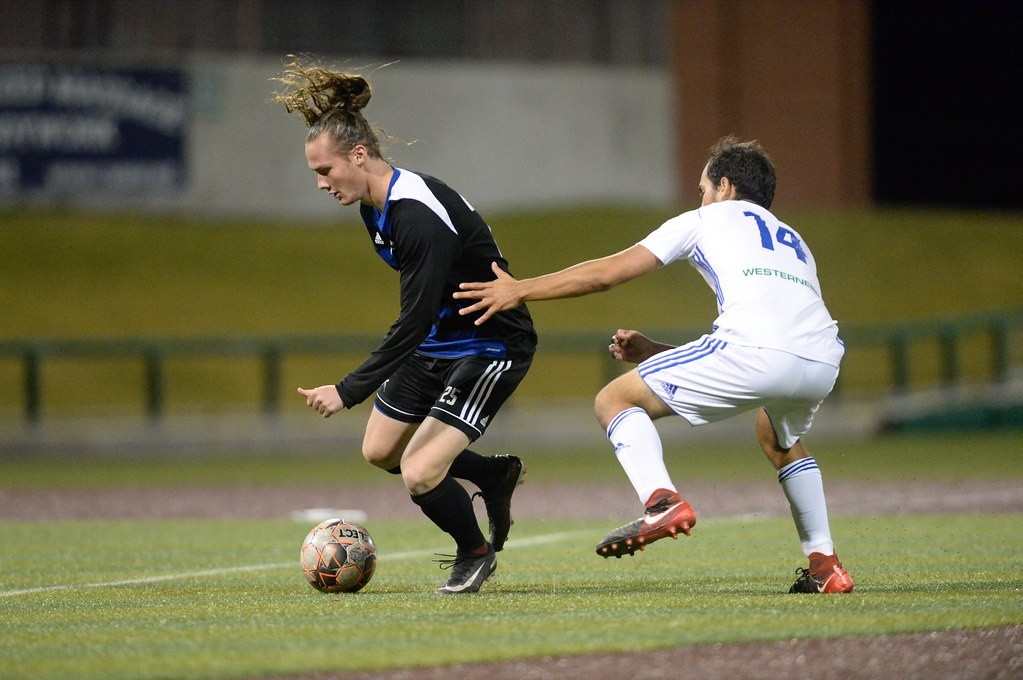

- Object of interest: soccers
[298,519,379,591]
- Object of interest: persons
[295,74,539,594]
[452,135,855,593]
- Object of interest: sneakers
[431,539,498,595]
[789,549,853,594]
[472,454,527,551]
[596,488,697,558]
